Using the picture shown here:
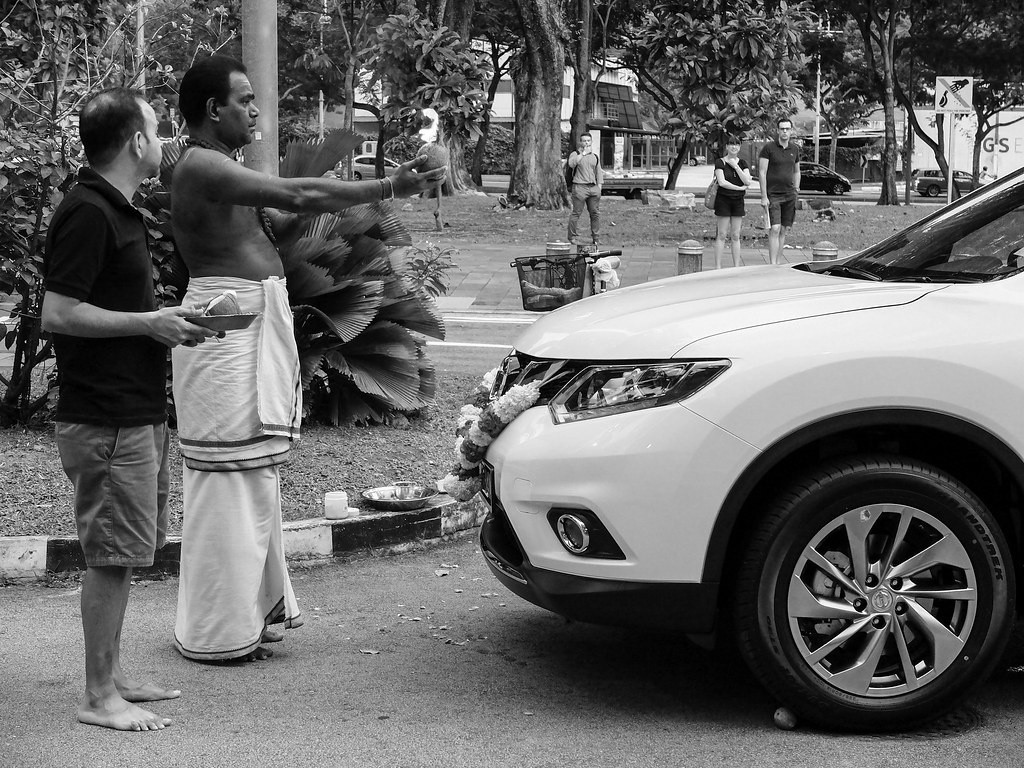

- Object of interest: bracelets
[379,175,394,203]
[793,187,799,190]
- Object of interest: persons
[567,132,604,246]
[759,118,801,265]
[714,136,752,270]
[40,85,226,733]
[980,166,994,180]
[169,54,449,665]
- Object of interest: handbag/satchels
[704,157,726,209]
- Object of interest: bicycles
[508,250,623,311]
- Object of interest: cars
[683,153,706,167]
[349,156,418,180]
[633,143,680,167]
[912,169,974,197]
[473,170,1024,737]
[797,162,853,196]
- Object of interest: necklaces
[724,156,739,177]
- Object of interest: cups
[325,492,349,519]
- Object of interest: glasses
[779,128,791,130]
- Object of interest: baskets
[515,254,587,311]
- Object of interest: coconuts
[204,292,241,315]
[414,140,448,180]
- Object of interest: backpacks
[564,149,599,193]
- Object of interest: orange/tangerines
[773,707,795,729]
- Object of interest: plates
[362,486,439,510]
[185,311,261,331]
